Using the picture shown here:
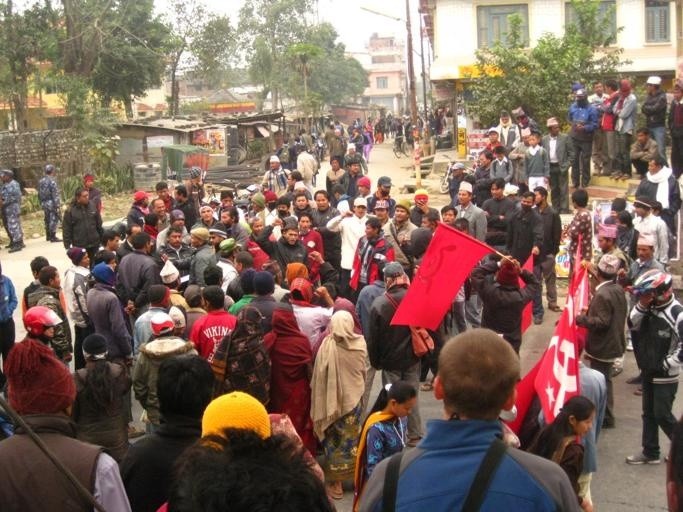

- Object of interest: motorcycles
[314,137,324,160]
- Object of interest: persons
[1,170,26,254]
[38,164,64,242]
[62,187,106,269]
[83,174,102,214]
[350,74,683,510]
[1,246,435,510]
[271,120,348,187]
[88,149,442,282]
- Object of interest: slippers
[420,383,432,391]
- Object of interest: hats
[190,167,201,179]
[45,165,53,172]
[270,155,280,163]
[190,222,242,253]
[647,76,661,85]
[134,191,152,201]
[5,340,77,414]
[201,392,271,448]
[547,117,558,128]
[149,260,187,336]
[0,170,13,177]
[383,261,403,277]
[488,107,529,137]
[451,163,472,193]
[241,269,274,294]
[354,176,427,213]
[598,196,653,274]
[170,210,185,225]
[252,191,277,209]
[67,248,114,285]
[497,260,518,286]
[82,334,109,361]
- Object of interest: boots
[5,239,25,252]
[46,231,62,242]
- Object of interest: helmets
[631,268,673,306]
[24,306,63,340]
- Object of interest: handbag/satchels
[412,328,435,357]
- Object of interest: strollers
[590,200,633,265]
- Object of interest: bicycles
[440,154,457,194]
[392,136,411,159]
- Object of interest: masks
[521,203,532,212]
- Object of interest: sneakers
[626,452,660,464]
